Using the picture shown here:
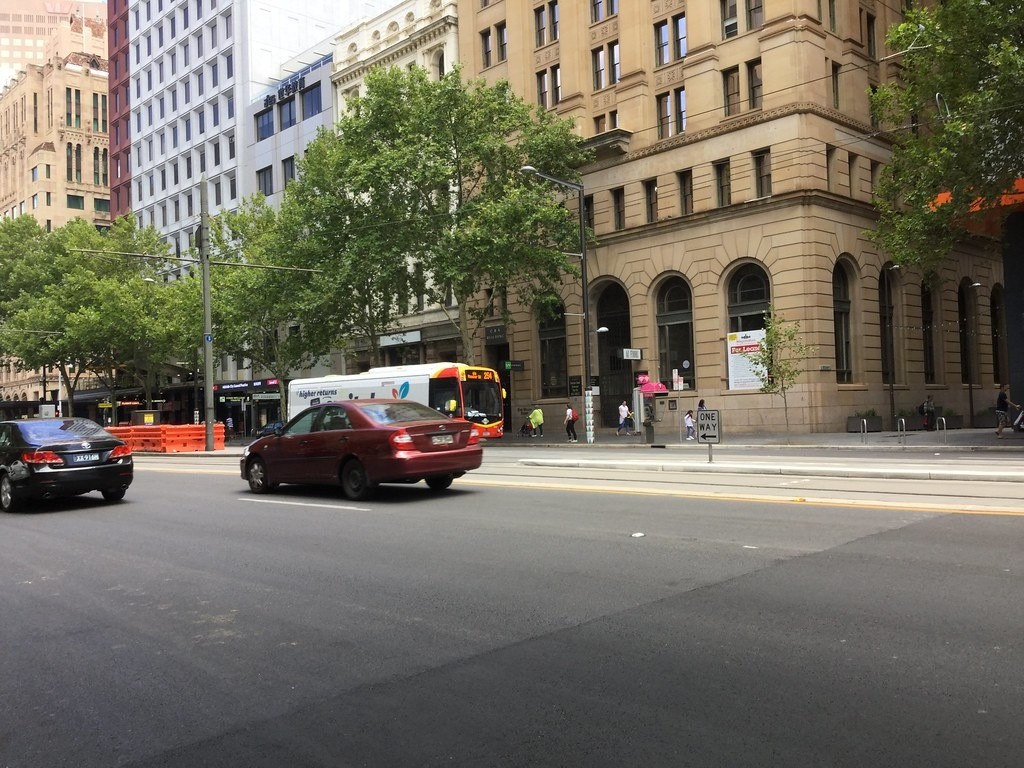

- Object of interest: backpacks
[919,400,928,415]
[572,409,578,422]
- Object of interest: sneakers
[540,434,543,438]
[566,439,578,443]
[532,435,538,437]
[686,436,694,441]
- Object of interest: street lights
[522,165,596,441]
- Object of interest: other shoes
[626,433,631,436]
[615,432,619,437]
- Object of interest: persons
[615,400,634,437]
[200,417,224,425]
[695,399,708,421]
[995,382,1022,440]
[225,413,234,441]
[526,404,545,438]
[684,410,697,442]
[563,404,578,443]
[923,393,937,432]
[445,394,457,412]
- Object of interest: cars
[0,415,134,511]
[239,397,483,498]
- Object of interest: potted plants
[847,407,883,432]
[942,407,964,429]
[898,408,927,430]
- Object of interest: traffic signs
[697,410,720,443]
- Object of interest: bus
[281,360,508,442]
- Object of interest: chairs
[236,422,244,438]
